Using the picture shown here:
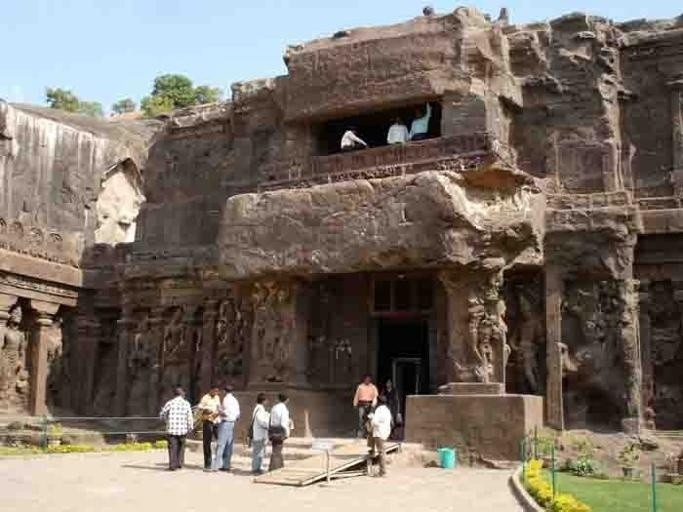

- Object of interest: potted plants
[616,445,641,481]
[46,421,62,448]
[537,434,560,469]
[194,410,204,440]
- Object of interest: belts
[359,401,372,403]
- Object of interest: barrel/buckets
[437,448,456,468]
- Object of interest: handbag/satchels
[396,413,402,427]
[248,426,252,439]
[269,426,285,441]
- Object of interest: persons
[386,114,410,144]
[354,375,379,439]
[160,387,195,470]
[251,392,272,472]
[365,394,392,477]
[409,102,432,140]
[339,125,369,151]
[379,377,400,439]
[193,385,220,471]
[269,393,295,471]
[202,385,241,472]
